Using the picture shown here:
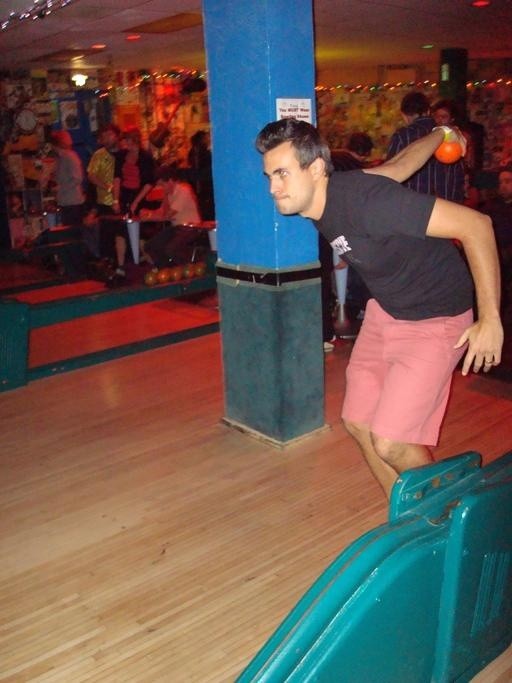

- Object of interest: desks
[100,214,170,266]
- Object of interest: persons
[386,91,474,205]
[329,132,373,171]
[0,113,222,285]
[256,112,504,504]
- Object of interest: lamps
[72,74,88,87]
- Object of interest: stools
[190,237,208,264]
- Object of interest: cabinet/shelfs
[6,186,62,249]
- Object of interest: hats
[49,130,73,150]
